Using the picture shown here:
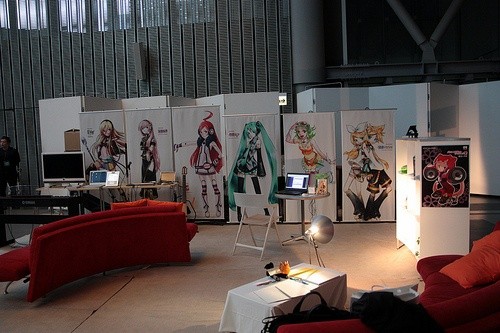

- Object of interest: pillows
[438,229,500,289]
[110,198,147,209]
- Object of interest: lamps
[404,124,419,138]
[305,214,335,268]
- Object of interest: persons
[0,136,20,210]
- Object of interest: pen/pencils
[302,281,308,285]
[257,281,272,286]
[280,261,284,266]
[287,260,288,264]
[285,261,286,266]
[290,278,298,279]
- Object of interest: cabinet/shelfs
[395,136,471,259]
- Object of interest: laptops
[275,172,310,195]
[83,171,107,188]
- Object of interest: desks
[219,263,348,333]
[35,182,180,216]
[275,192,331,248]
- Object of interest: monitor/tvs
[41,152,85,186]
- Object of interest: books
[288,266,339,285]
[318,179,328,193]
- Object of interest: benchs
[0,200,199,303]
[276,252,500,333]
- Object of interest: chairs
[232,191,281,262]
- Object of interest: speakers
[278,176,285,191]
[133,42,146,79]
